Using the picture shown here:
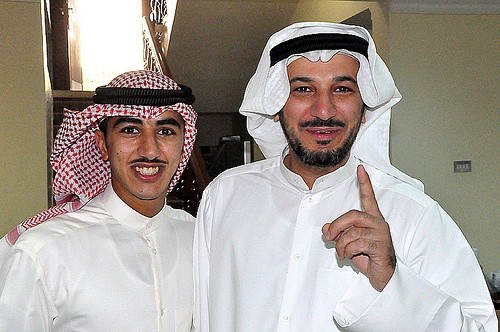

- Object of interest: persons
[0,70,197,332]
[195,21,499,332]
